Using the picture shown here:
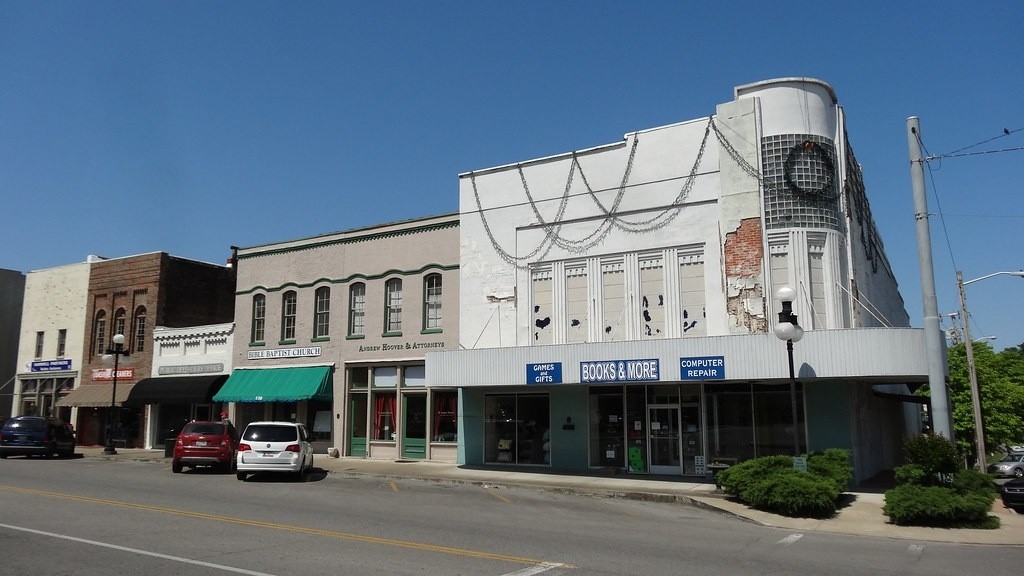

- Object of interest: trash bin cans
[164,438,177,457]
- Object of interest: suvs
[171,419,239,474]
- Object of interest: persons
[221,412,238,457]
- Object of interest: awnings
[55,384,136,407]
[125,374,229,406]
[212,367,330,402]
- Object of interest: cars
[1000,474,1024,514]
[1007,444,1020,451]
[405,413,457,442]
[0,413,78,459]
[987,452,1024,477]
[236,422,318,480]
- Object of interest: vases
[327,448,337,458]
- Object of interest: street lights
[956,270,1024,473]
[101,334,132,454]
[774,286,804,457]
